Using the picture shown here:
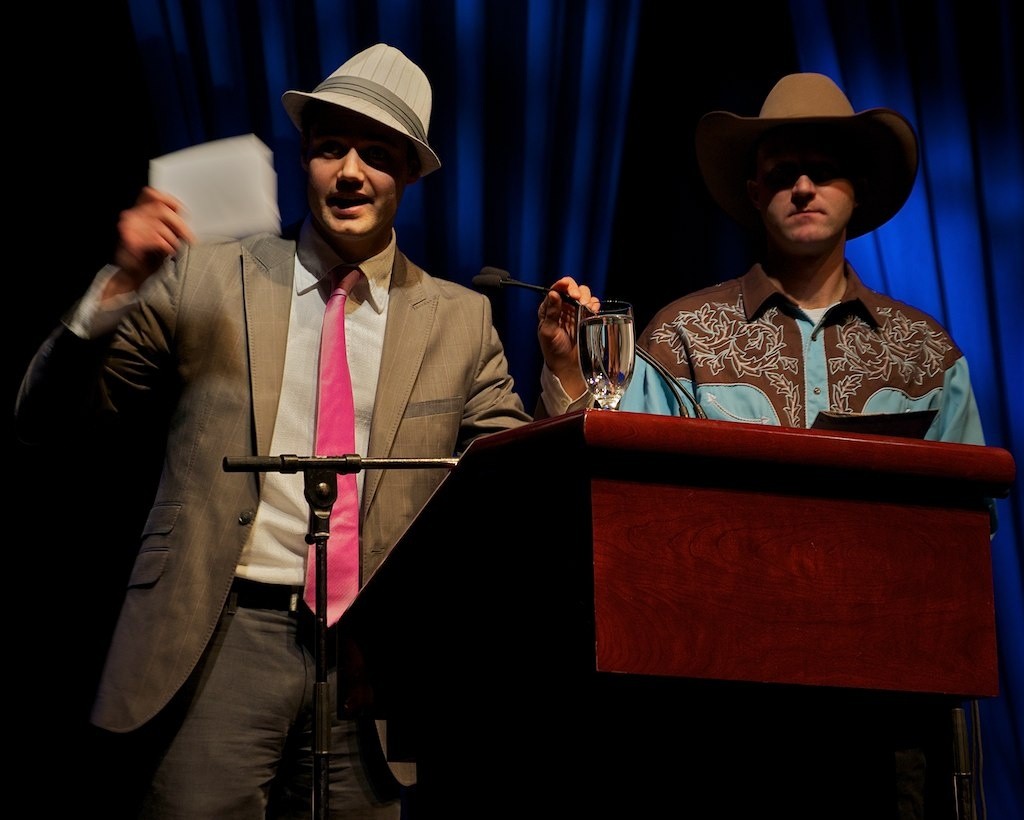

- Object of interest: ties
[302,268,358,630]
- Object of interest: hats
[280,43,441,177]
[695,73,919,241]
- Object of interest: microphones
[472,267,706,420]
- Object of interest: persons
[618,71,986,445]
[5,40,607,820]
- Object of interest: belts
[225,578,304,609]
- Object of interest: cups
[577,301,636,409]
